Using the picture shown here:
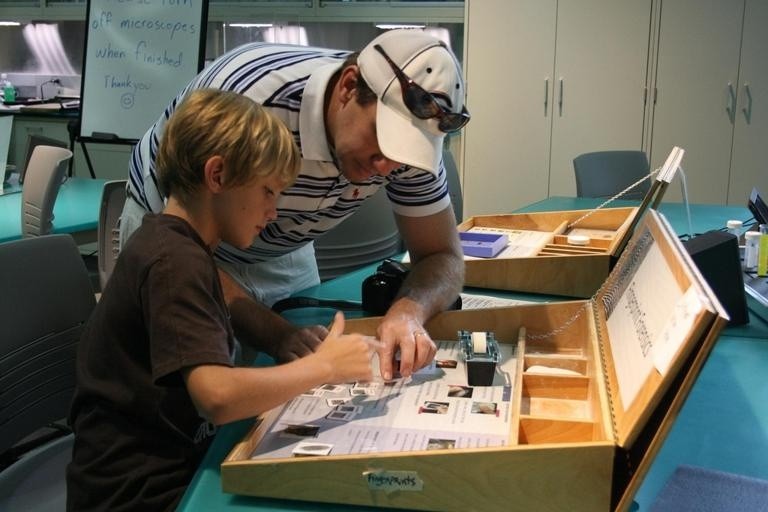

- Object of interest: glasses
[373,43,471,134]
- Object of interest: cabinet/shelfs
[73,144,133,180]
[14,119,72,175]
[649,0,768,208]
[461,0,653,222]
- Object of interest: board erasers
[91,131,118,142]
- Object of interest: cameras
[363,259,462,313]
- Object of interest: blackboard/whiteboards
[80,1,202,141]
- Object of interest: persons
[66,88,376,510]
[115,25,472,380]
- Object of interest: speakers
[682,230,749,327]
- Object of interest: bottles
[0,74,15,103]
[757,223,768,277]
[725,220,744,242]
[742,231,759,274]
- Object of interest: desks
[0,110,97,179]
[0,178,116,242]
[175,195,768,512]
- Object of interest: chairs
[314,186,406,283]
[97,180,129,294]
[0,235,97,512]
[21,145,73,237]
[574,151,651,204]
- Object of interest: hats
[356,28,465,181]
